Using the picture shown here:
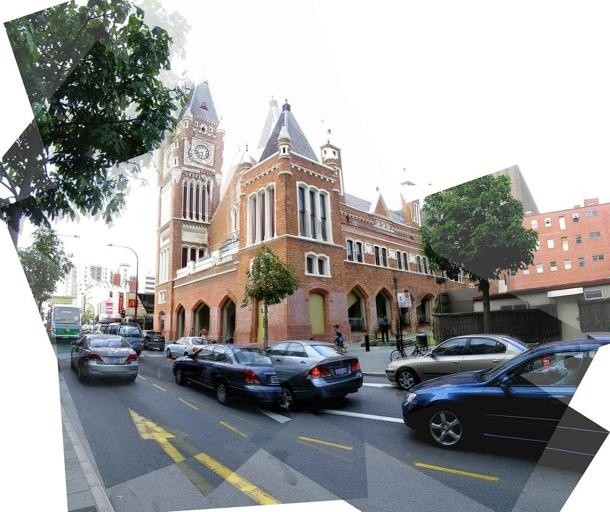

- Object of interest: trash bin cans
[416,332,428,351]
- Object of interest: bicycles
[390,344,428,362]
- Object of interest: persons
[188,327,194,337]
[199,327,208,338]
[332,325,348,354]
[535,356,578,386]
[381,315,390,344]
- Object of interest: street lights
[106,244,138,323]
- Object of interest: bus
[48,304,82,344]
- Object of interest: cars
[166,337,207,358]
[402,338,610,448]
[71,322,165,381]
[172,340,363,411]
[385,334,531,389]
